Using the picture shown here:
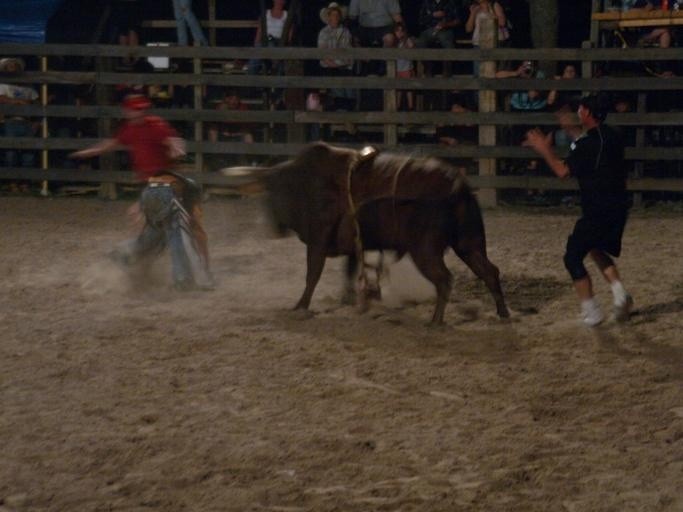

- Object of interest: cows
[219,141,510,329]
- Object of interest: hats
[320,2,349,25]
[122,95,153,110]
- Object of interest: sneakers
[565,307,605,329]
[609,290,633,324]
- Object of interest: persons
[64,92,205,222]
[518,93,634,327]
[0,1,682,201]
